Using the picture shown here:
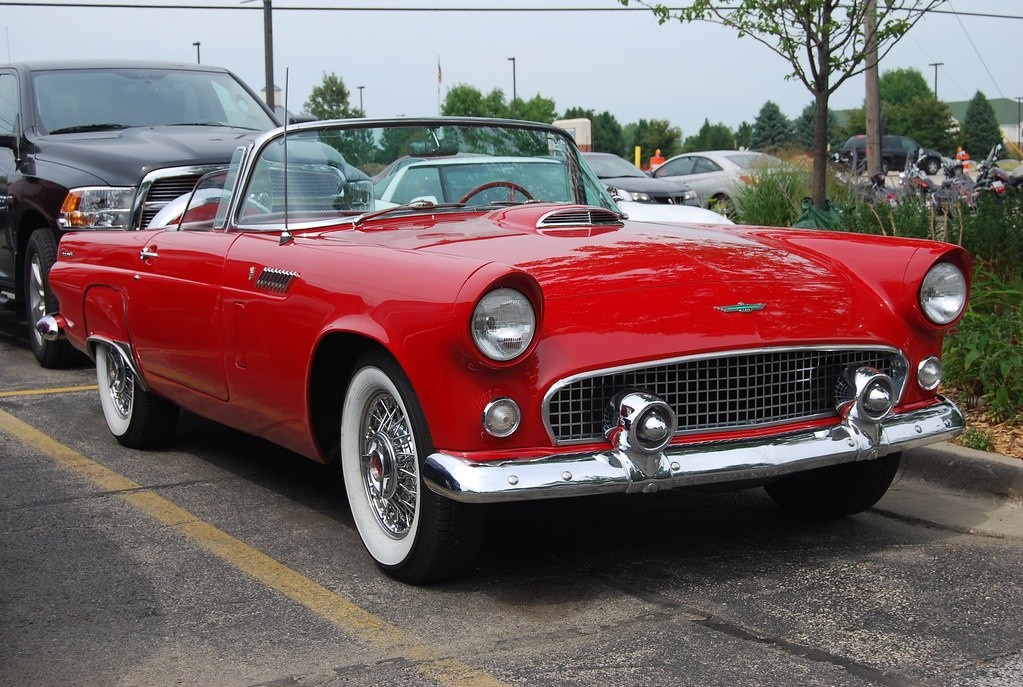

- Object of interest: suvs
[0,57,375,369]
[841,134,943,177]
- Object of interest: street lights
[193,41,200,63]
[356,85,365,117]
[1014,96,1023,161]
[508,57,518,122]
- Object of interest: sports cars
[48,116,975,582]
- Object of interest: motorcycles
[826,142,1023,219]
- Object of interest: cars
[645,150,808,219]
[579,151,701,215]
